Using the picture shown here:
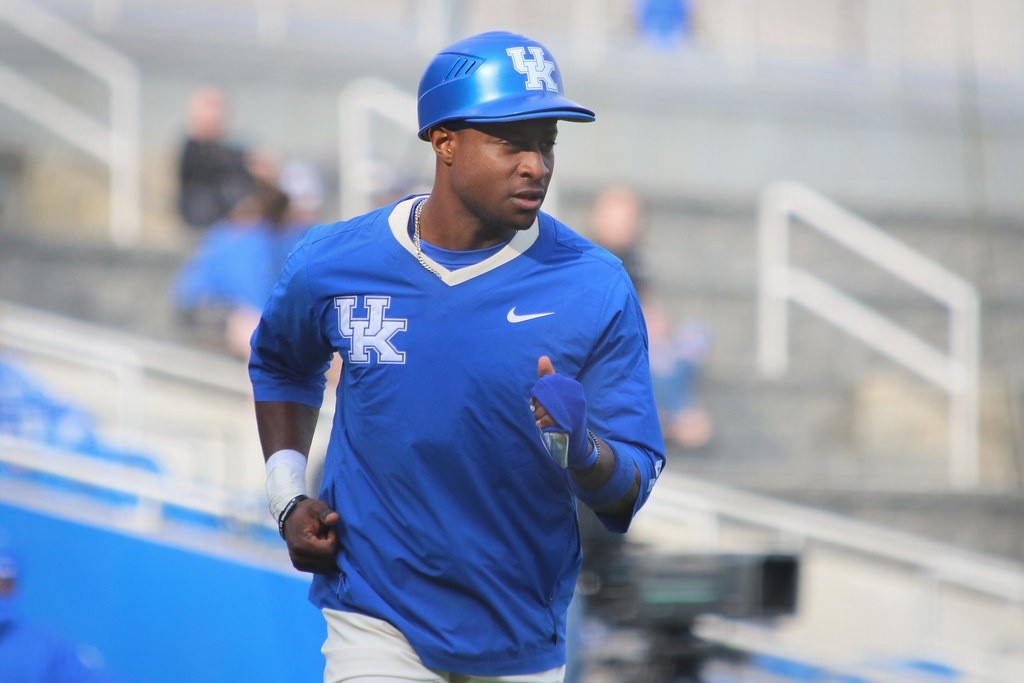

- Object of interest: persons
[165,81,715,458]
[247,31,667,683]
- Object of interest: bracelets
[571,428,600,472]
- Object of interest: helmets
[417,31,595,146]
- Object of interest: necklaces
[411,199,518,280]
